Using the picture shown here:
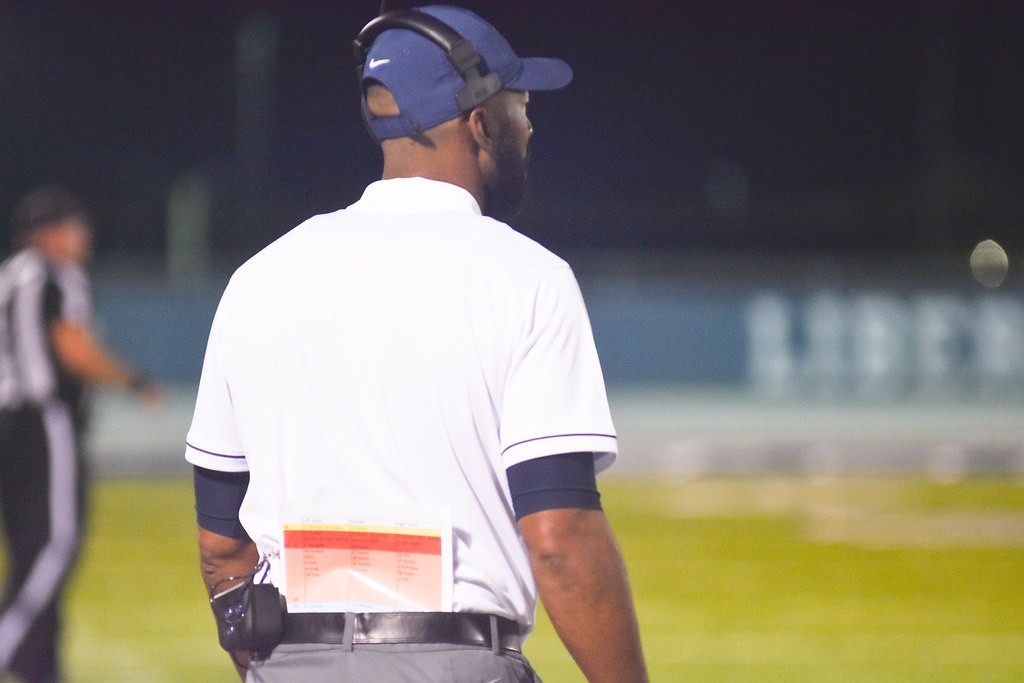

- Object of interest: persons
[0,185,169,683]
[184,5,648,683]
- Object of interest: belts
[277,612,522,654]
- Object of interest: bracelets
[130,372,148,391]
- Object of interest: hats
[360,5,573,140]
